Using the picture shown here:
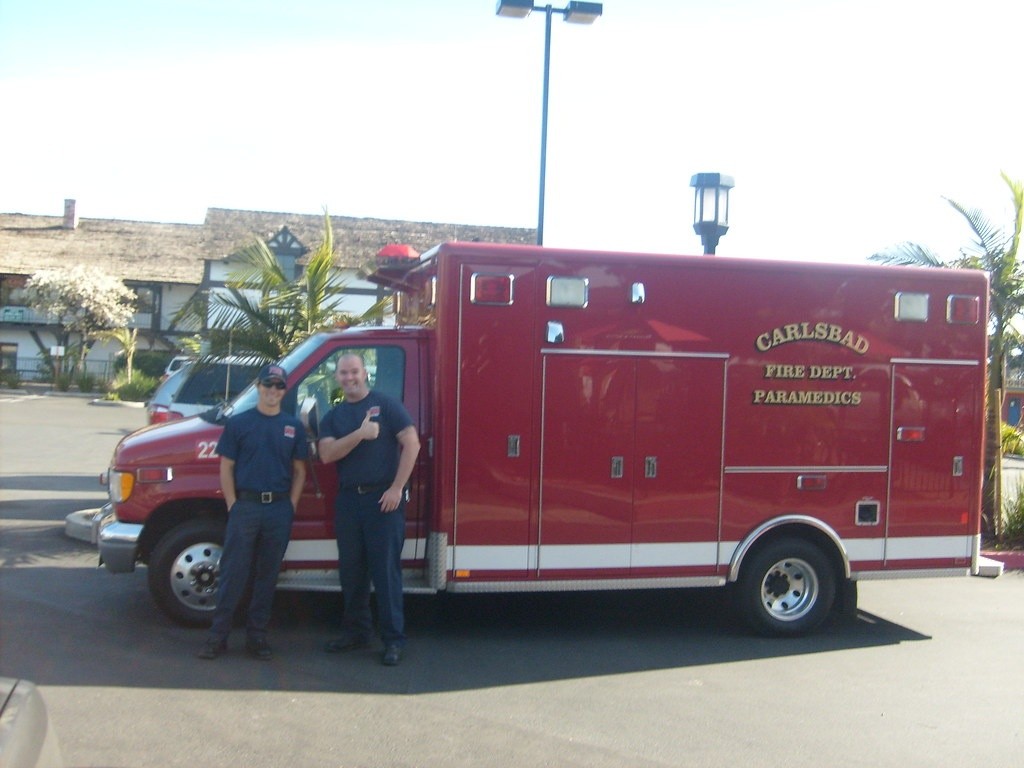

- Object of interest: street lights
[494,0,605,246]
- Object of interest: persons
[316,355,423,667]
[199,364,311,661]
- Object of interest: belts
[340,483,375,494]
[235,490,286,505]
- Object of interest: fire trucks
[98,241,1005,644]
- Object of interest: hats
[258,365,287,387]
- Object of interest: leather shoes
[245,636,272,659]
[325,633,372,653]
[383,644,404,665]
[198,637,228,659]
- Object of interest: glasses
[262,380,285,390]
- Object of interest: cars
[143,354,305,429]
[159,354,192,389]
